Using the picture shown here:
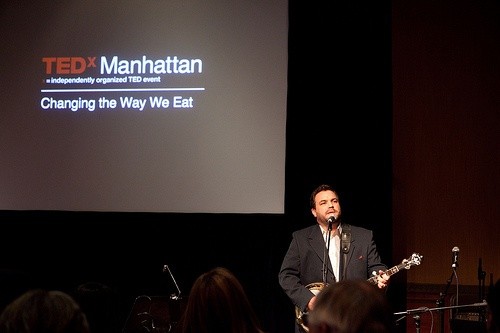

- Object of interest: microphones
[452,246,460,269]
[163,264,167,272]
[328,216,335,230]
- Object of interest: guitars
[295,253,424,333]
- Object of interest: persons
[0,288,91,333]
[278,184,391,333]
[179,266,264,333]
[308,278,396,333]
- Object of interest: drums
[128,293,189,333]
